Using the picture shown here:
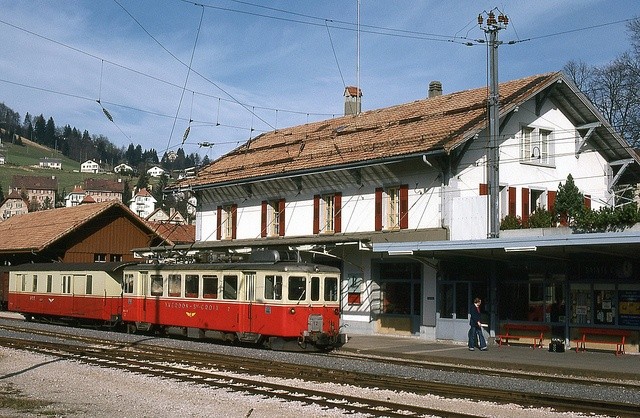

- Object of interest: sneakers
[481,346,487,350]
[469,347,475,351]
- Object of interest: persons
[467,297,488,351]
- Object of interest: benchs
[496,323,549,350]
[572,327,631,356]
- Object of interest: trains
[5,242,349,354]
